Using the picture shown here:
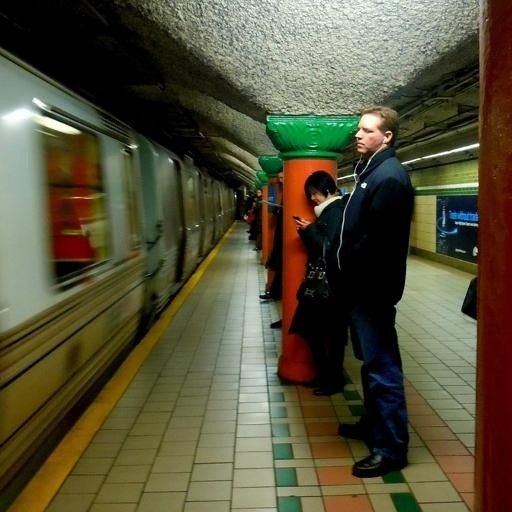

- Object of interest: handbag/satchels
[296,237,332,302]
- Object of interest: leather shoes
[350,452,409,479]
[271,320,284,329]
[304,380,322,388]
[247,229,260,251]
[313,386,345,396]
[259,291,275,300]
[337,422,374,441]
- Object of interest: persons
[287,170,350,395]
[328,106,415,478]
[242,165,285,300]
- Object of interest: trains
[0,45,240,497]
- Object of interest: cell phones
[293,216,301,221]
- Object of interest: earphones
[383,136,387,144]
